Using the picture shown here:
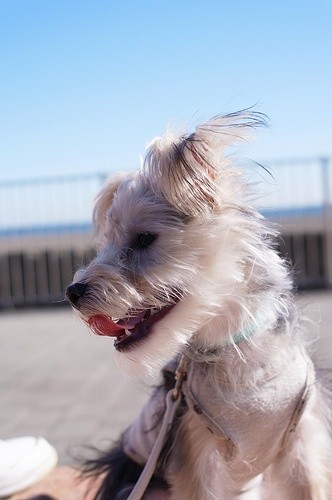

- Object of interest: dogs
[66,103,332,499]
